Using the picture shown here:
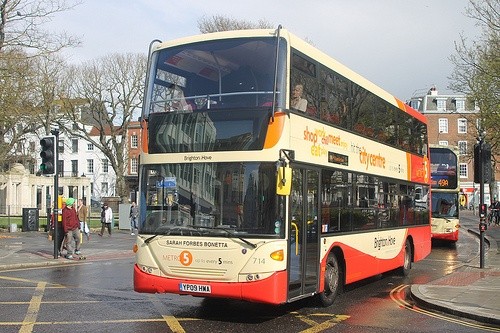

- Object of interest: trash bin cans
[22,208,39,231]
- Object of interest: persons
[488,198,500,227]
[75,199,92,240]
[49,198,68,256]
[63,198,81,258]
[98,203,113,237]
[290,84,308,113]
[129,201,138,236]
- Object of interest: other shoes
[58,252,60,257]
[88,235,91,240]
[98,233,103,237]
[75,250,82,255]
[131,232,135,235]
[108,233,112,236]
[66,253,73,259]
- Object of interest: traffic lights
[39,137,57,176]
[478,203,487,218]
[479,223,486,231]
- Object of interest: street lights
[437,130,443,145]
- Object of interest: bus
[428,143,467,244]
[132,25,434,309]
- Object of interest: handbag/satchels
[49,228,55,240]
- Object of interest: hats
[104,203,108,206]
[66,198,74,206]
